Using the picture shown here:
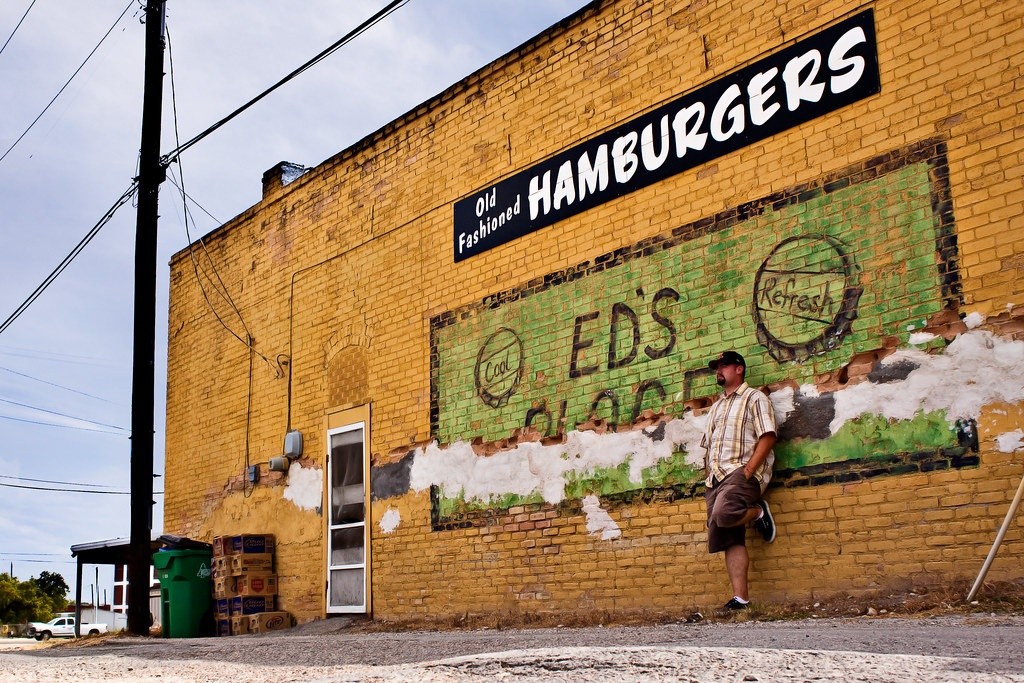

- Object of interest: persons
[700,351,778,612]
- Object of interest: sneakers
[716,597,756,616]
[753,500,779,545]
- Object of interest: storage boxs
[211,534,290,637]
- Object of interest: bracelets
[748,469,753,474]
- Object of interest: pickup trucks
[28,616,107,641]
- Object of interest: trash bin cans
[152,535,212,638]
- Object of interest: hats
[708,350,750,368]
